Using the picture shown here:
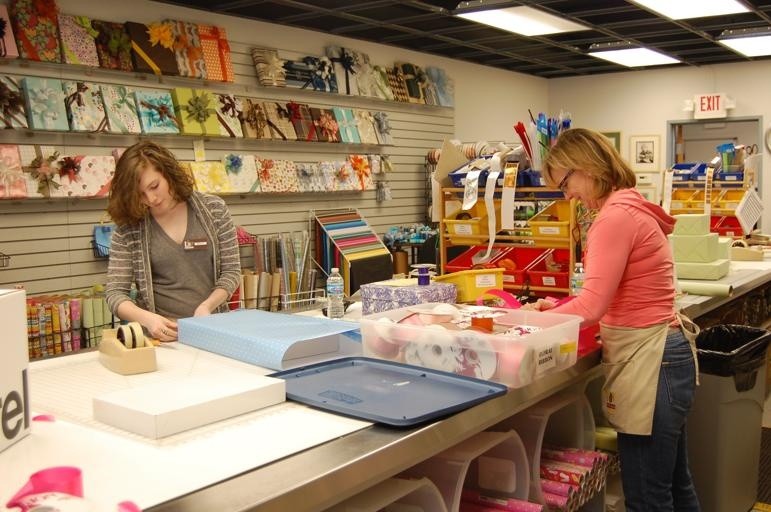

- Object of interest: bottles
[572,262,584,293]
[326,267,345,318]
[399,227,433,244]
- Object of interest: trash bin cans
[686,324,771,512]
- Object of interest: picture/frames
[627,133,662,204]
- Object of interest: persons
[102,136,242,342]
[518,127,702,510]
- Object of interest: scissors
[746,144,758,156]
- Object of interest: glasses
[556,169,575,193]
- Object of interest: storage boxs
[0,0,457,201]
[663,162,746,238]
[360,161,585,390]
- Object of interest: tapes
[731,240,747,247]
[115,321,146,349]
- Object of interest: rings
[162,328,168,335]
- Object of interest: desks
[30,245,770,512]
